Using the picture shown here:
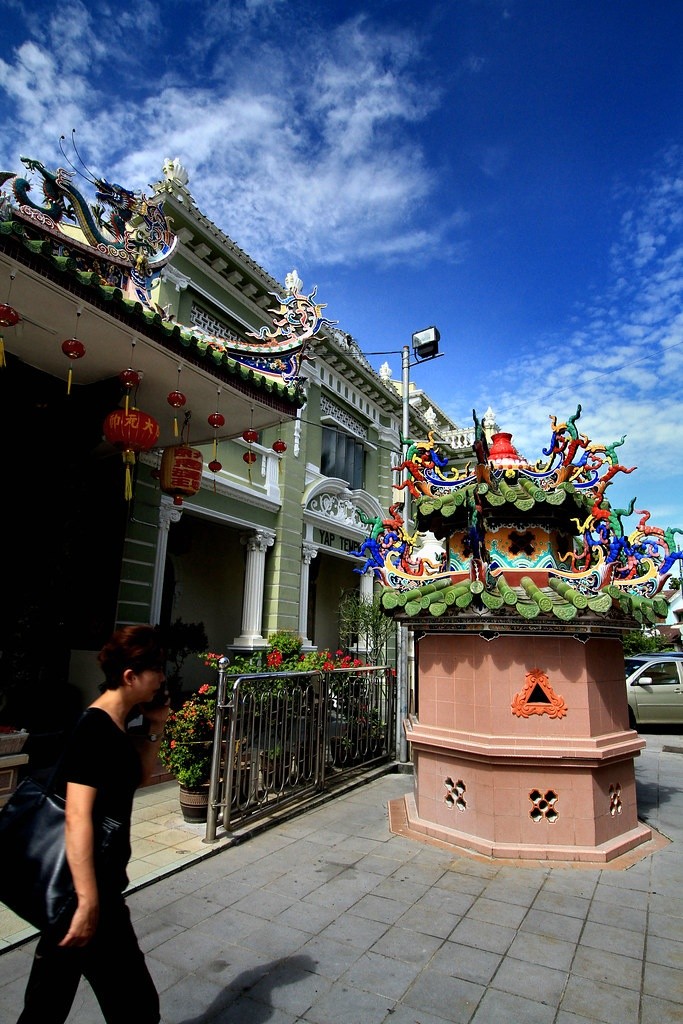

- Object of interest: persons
[4,617,182,1024]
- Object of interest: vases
[175,777,224,825]
[216,761,254,810]
[258,734,386,788]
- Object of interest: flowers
[156,684,222,789]
[204,645,398,736]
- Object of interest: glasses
[148,663,166,673]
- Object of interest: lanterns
[209,412,227,464]
[60,336,87,397]
[238,451,258,488]
[115,368,141,419]
[242,426,258,467]
[0,303,20,368]
[272,438,289,476]
[163,391,186,439]
[209,460,224,496]
[101,407,163,504]
[158,445,203,507]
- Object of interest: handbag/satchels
[0,709,123,940]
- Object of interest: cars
[637,651,683,658]
[618,655,683,732]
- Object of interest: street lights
[399,324,443,774]
